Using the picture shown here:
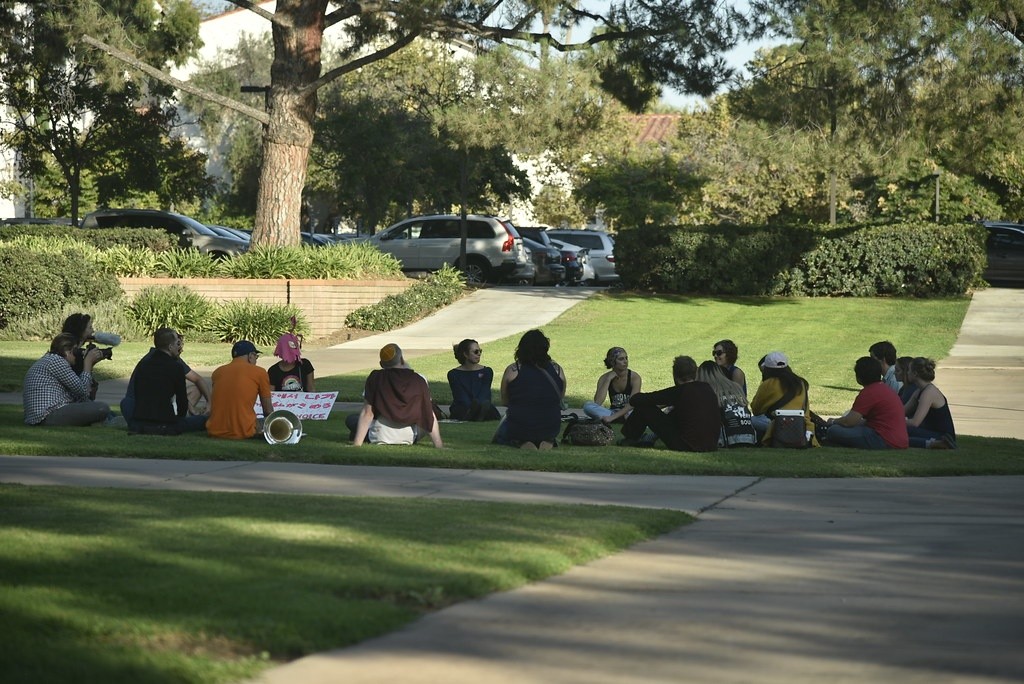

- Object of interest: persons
[750,351,822,448]
[583,347,642,424]
[447,339,501,421]
[346,344,446,449]
[205,340,274,440]
[23,331,127,427]
[617,355,721,452]
[268,333,318,392]
[697,360,757,449]
[712,339,748,398]
[904,357,956,440]
[869,341,904,395]
[120,327,212,436]
[826,357,909,450]
[893,356,916,405]
[493,329,567,449]
[810,411,957,449]
[61,313,103,402]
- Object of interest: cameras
[73,344,113,362]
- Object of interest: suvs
[363,212,528,288]
[77,208,250,264]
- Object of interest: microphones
[88,331,121,347]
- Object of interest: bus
[545,228,621,286]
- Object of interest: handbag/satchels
[771,415,808,449]
[562,418,616,447]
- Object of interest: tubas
[263,410,308,445]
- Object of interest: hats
[380,345,397,362]
[232,340,263,358]
[760,352,787,368]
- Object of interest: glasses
[177,341,181,347]
[252,353,259,359]
[712,350,727,356]
[469,348,483,354]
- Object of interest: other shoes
[943,433,958,450]
[539,438,555,449]
[91,415,128,430]
[640,432,657,448]
[617,437,644,448]
[513,439,537,450]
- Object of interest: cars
[204,221,362,251]
[977,227,1024,282]
[514,224,598,286]
